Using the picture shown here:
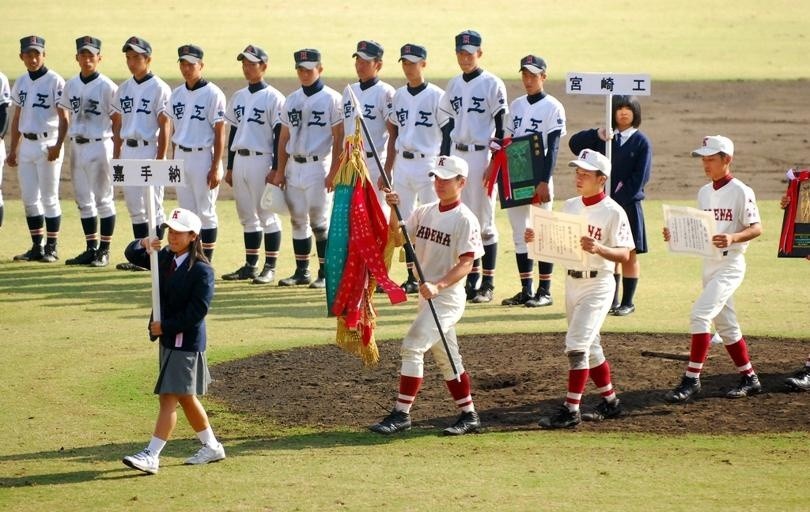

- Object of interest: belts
[451,141,485,153]
[69,133,107,145]
[566,268,602,280]
[722,249,732,257]
[287,151,320,166]
[363,149,378,158]
[393,146,428,161]
[232,146,267,159]
[121,134,150,149]
[178,144,209,154]
[18,130,51,140]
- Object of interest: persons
[8,36,66,262]
[489,56,567,307]
[524,149,636,431]
[274,48,343,288]
[0,70,10,227]
[435,30,508,303]
[367,155,486,435]
[122,207,226,476]
[222,45,286,284]
[162,44,226,265]
[342,41,396,293]
[111,37,172,272]
[569,96,653,315]
[663,136,764,404]
[56,36,119,267]
[777,196,810,393]
[377,43,450,295]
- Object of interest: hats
[293,48,322,70]
[397,43,428,64]
[690,134,735,161]
[518,55,546,77]
[454,29,482,59]
[352,40,383,61]
[121,35,152,56]
[158,207,202,235]
[176,45,203,64]
[75,35,101,56]
[236,44,268,65]
[567,148,614,178]
[19,35,46,53]
[428,156,469,181]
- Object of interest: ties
[614,133,626,154]
[161,257,179,284]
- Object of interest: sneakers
[374,284,383,293]
[471,282,494,304]
[586,398,624,419]
[779,365,810,393]
[464,285,476,300]
[117,261,145,271]
[252,263,276,283]
[525,286,554,308]
[611,302,619,311]
[537,405,582,429]
[614,304,635,316]
[442,411,482,435]
[278,269,311,287]
[663,375,702,404]
[502,292,529,306]
[65,249,95,266]
[39,244,59,263]
[308,269,327,289]
[725,373,762,399]
[90,249,110,266]
[398,280,420,294]
[13,247,42,261]
[121,450,159,476]
[367,408,411,434]
[182,443,226,466]
[221,264,256,281]
